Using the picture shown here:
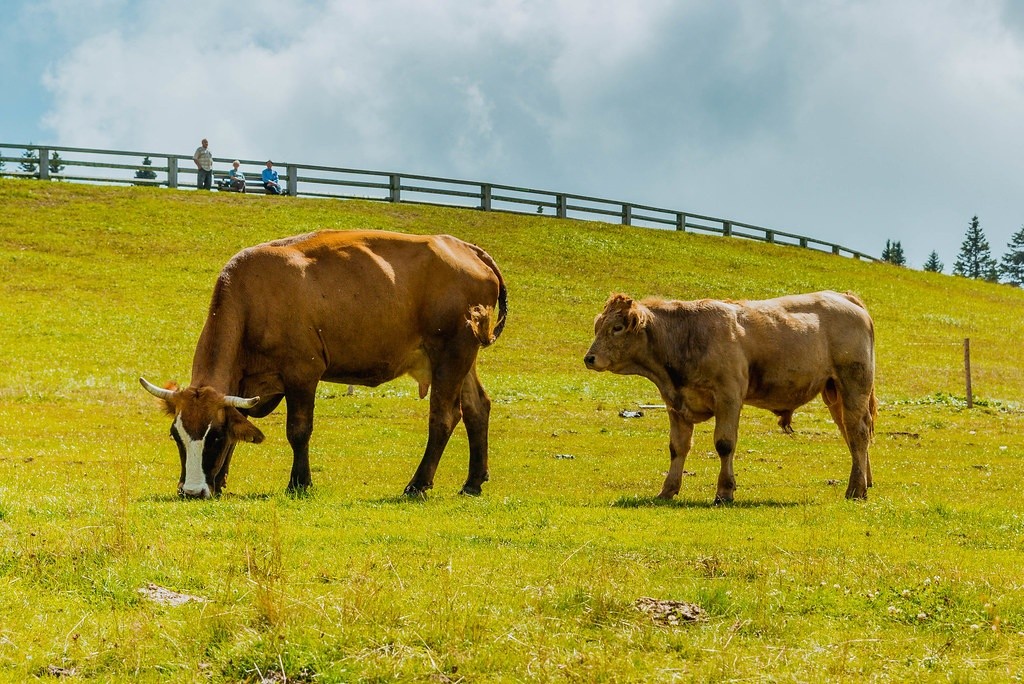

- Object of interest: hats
[266,160,273,164]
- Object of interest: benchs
[214,174,273,194]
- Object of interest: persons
[262,161,281,195]
[229,161,246,193]
[193,139,212,190]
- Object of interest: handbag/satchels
[218,178,228,187]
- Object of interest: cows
[138,228,510,503]
[584,290,880,506]
